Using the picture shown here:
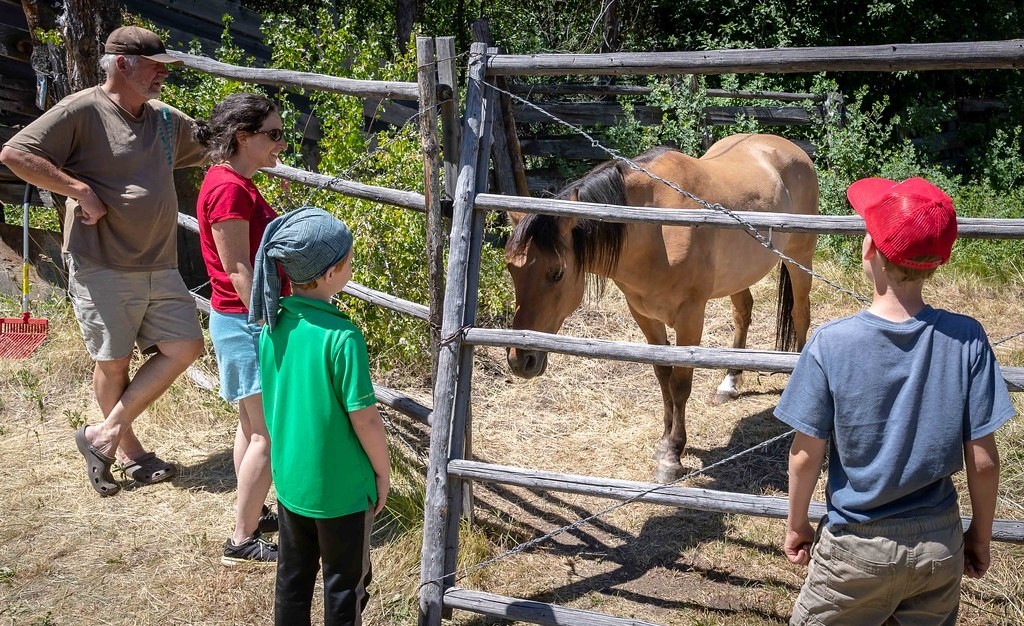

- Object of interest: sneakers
[221,527,282,570]
[255,502,281,532]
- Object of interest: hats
[103,24,185,71]
[847,176,960,272]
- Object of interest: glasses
[243,127,288,144]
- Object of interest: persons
[0,26,290,497]
[258,210,391,626]
[773,178,1019,626]
[192,93,291,566]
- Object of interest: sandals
[114,450,176,484]
[73,423,122,498]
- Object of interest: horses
[502,132,821,483]
